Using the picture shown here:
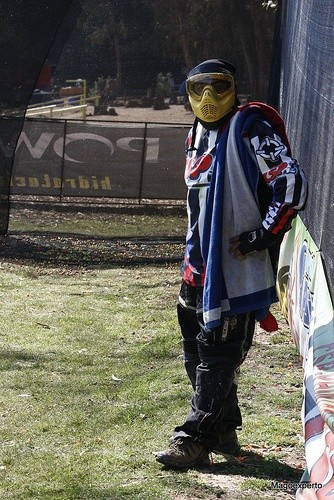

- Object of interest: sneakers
[212,431,244,455]
[160,430,212,467]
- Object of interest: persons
[154,60,310,469]
[167,72,176,104]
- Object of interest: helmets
[186,58,238,130]
